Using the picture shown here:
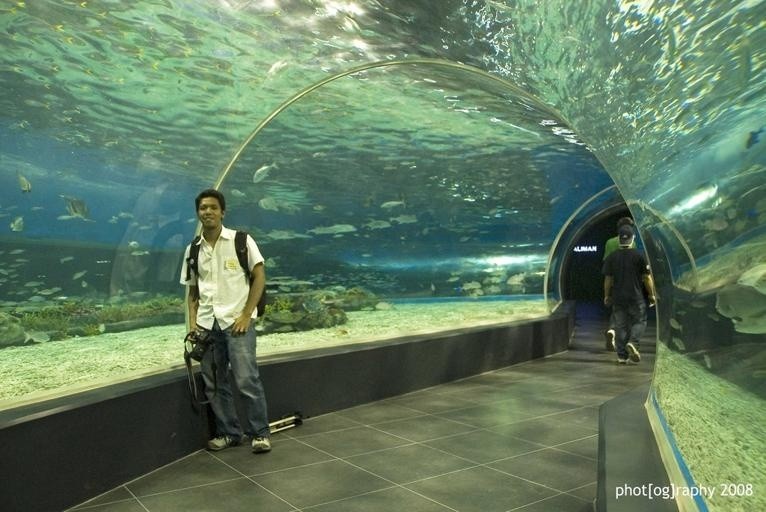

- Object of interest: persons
[600,216,637,352]
[176,188,272,455]
[601,224,656,365]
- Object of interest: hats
[617,217,635,246]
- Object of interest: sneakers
[625,343,641,362]
[208,435,239,451]
[617,356,629,363]
[252,436,271,452]
[606,329,617,351]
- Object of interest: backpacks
[185,229,267,317]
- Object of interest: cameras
[188,328,216,363]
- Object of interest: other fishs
[0,1,766,381]
